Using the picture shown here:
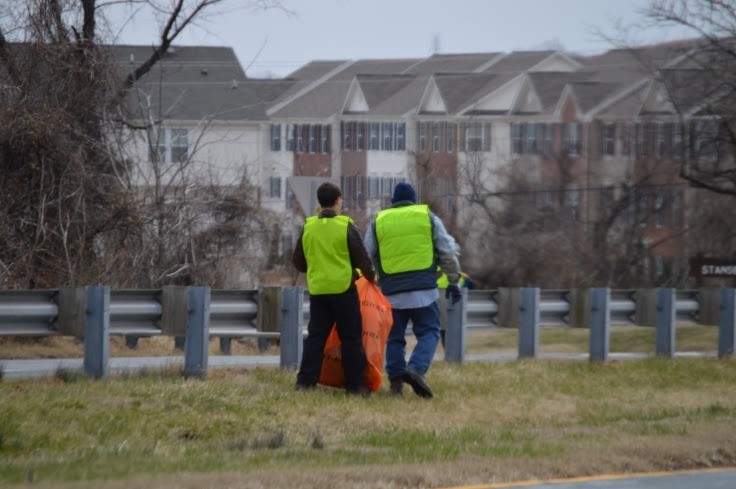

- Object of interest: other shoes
[403,370,432,398]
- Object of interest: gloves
[445,284,462,305]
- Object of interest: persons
[364,182,461,399]
[291,182,375,396]
[435,265,479,350]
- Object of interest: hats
[391,182,417,203]
